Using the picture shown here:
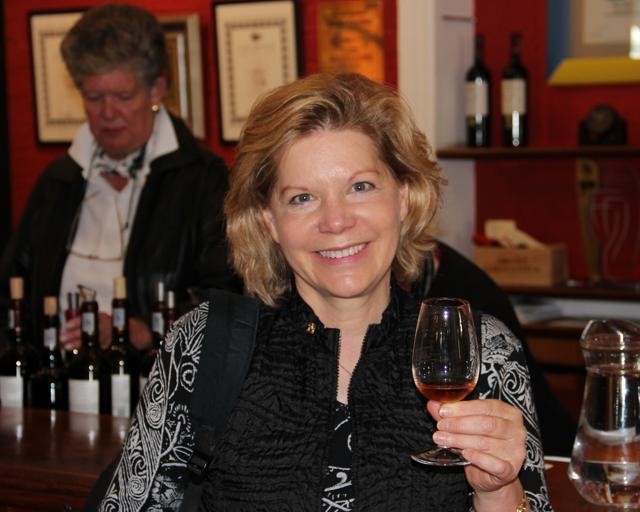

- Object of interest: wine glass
[409,296,481,468]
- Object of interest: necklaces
[339,361,352,378]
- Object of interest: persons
[99,73,556,511]
[13,7,246,376]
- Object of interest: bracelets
[515,490,528,511]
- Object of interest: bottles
[0,276,177,418]
[461,31,493,149]
[567,317,640,509]
[499,32,529,148]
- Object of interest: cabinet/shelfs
[435,143,640,368]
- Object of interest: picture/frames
[154,13,207,140]
[27,7,93,150]
[211,0,304,150]
[546,0,640,87]
